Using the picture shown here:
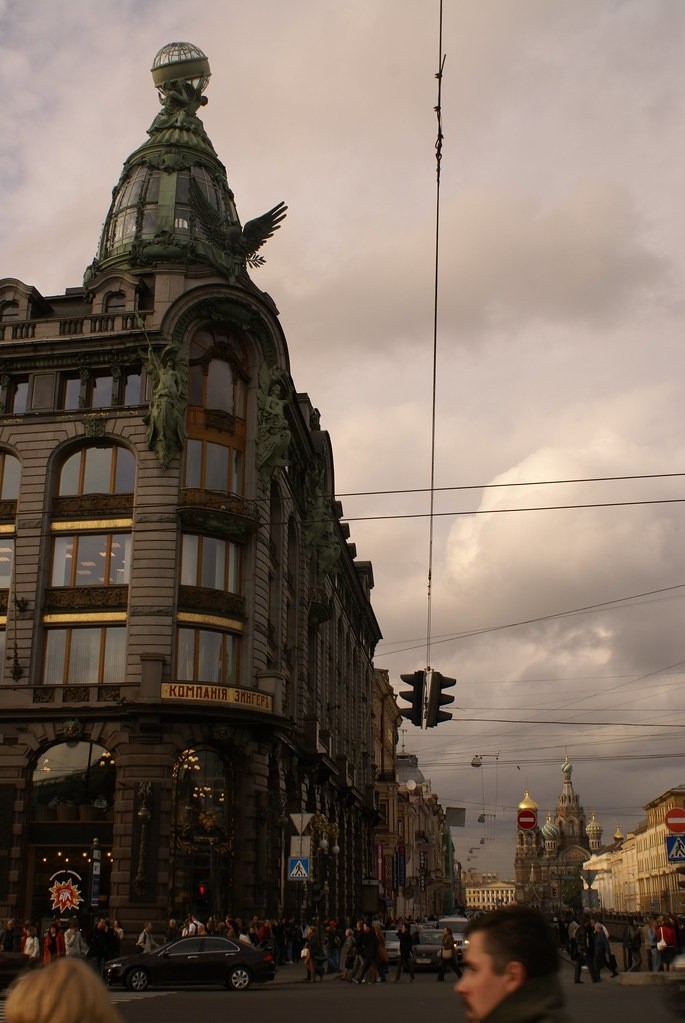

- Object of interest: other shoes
[594,977,605,982]
[575,980,584,984]
[353,978,359,983]
[611,972,619,977]
[391,978,400,982]
[406,978,416,983]
[305,977,311,980]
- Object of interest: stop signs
[517,810,538,830]
[665,807,685,833]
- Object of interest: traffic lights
[399,669,426,729]
[423,670,456,731]
[196,886,208,905]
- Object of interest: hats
[329,921,336,928]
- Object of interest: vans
[437,918,470,959]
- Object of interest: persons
[455,905,574,1023]
[438,927,463,981]
[625,914,685,972]
[166,913,389,984]
[146,79,208,132]
[391,923,415,983]
[265,385,293,466]
[0,917,124,967]
[560,916,619,983]
[312,468,340,571]
[143,344,189,468]
[6,958,123,1023]
[387,914,435,930]
[136,922,160,953]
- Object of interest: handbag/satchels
[345,957,355,970]
[436,950,441,958]
[35,952,42,962]
[442,949,453,959]
[300,947,310,959]
[609,953,617,971]
[135,946,144,953]
[409,954,418,964]
[656,939,667,952]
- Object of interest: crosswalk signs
[289,858,310,882]
[664,835,685,863]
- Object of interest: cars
[105,935,278,993]
[383,930,402,963]
[411,929,451,972]
